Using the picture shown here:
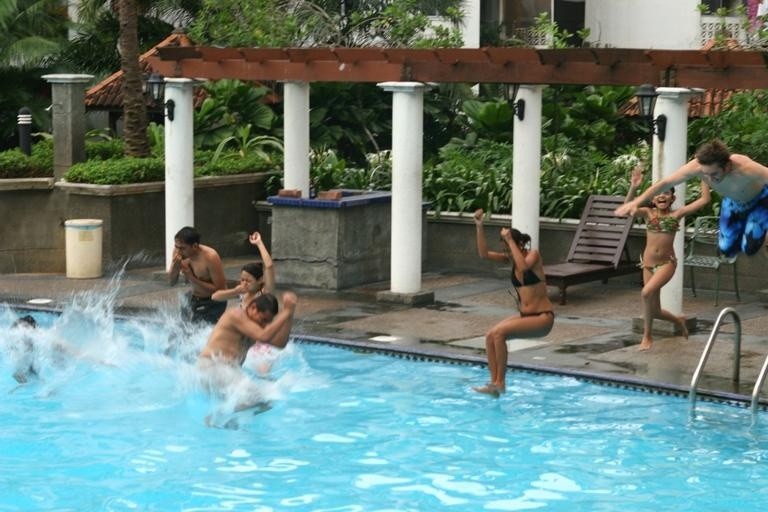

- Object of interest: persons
[169,226,226,326]
[200,290,298,428]
[472,208,554,397]
[615,138,768,254]
[11,315,43,379]
[624,167,712,351]
[212,232,276,364]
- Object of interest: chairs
[543,195,640,305]
[685,216,740,306]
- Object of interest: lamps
[145,70,175,121]
[635,83,667,142]
[501,83,525,121]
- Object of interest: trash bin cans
[65,219,102,279]
